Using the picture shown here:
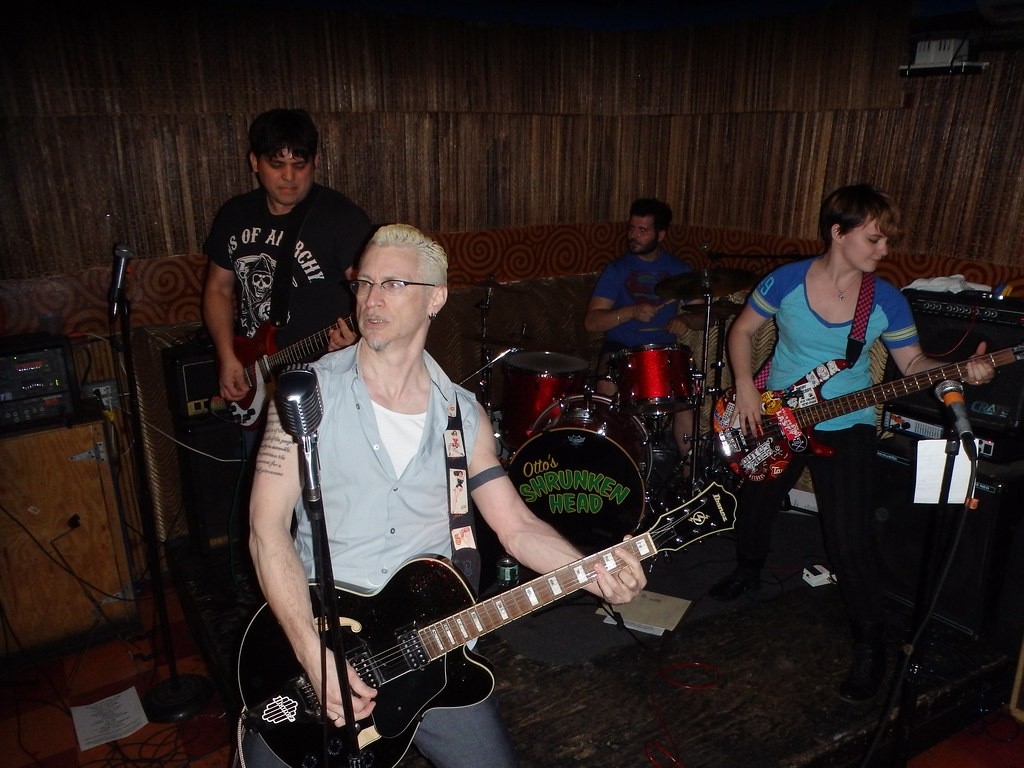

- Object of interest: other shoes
[708,572,759,601]
[840,639,883,700]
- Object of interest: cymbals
[681,305,744,317]
[461,332,556,351]
[655,268,761,301]
[450,278,538,298]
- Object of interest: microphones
[276,364,323,502]
[934,379,976,460]
[111,242,134,317]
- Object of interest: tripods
[655,275,717,515]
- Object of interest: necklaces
[820,259,862,300]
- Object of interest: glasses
[350,279,438,294]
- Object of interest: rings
[332,716,341,722]
[975,380,984,385]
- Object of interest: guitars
[712,342,1024,485]
[212,312,358,431]
[232,479,743,768]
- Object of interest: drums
[506,391,653,551]
[610,343,698,416]
[498,350,593,450]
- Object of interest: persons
[584,197,705,492]
[709,182,994,703]
[235,223,647,768]
[202,108,376,645]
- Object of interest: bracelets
[616,309,623,325]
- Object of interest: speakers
[172,413,242,586]
[875,288,1024,642]
